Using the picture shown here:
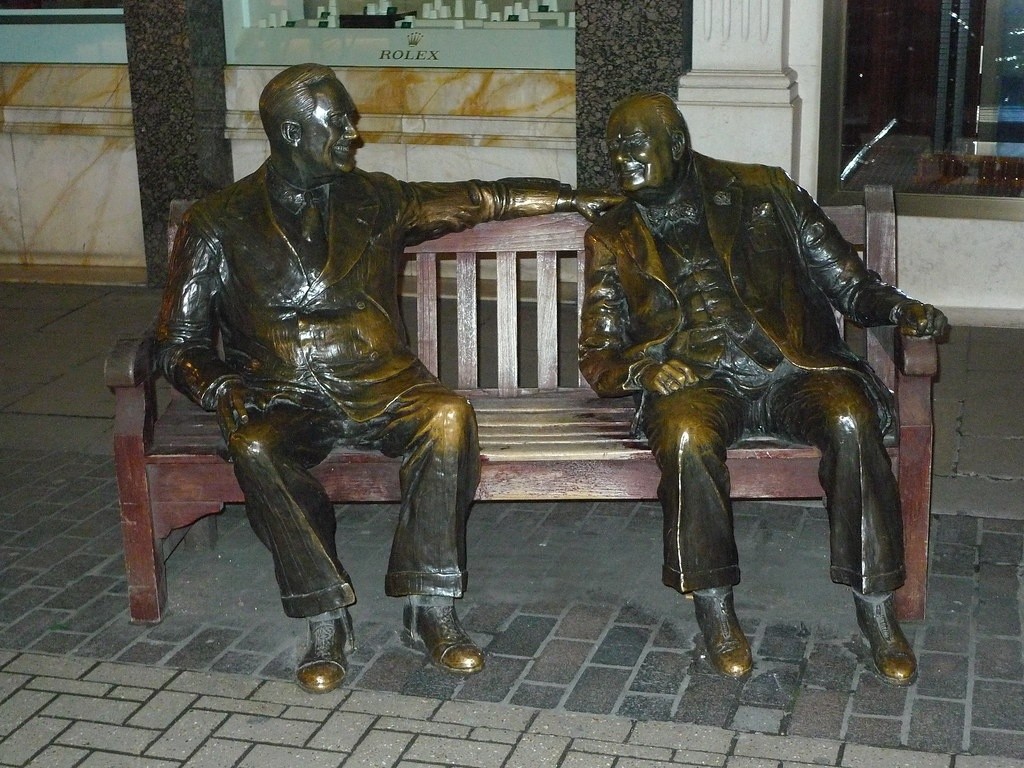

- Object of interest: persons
[151,63,627,695]
[577,91,948,685]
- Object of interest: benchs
[101,186,939,622]
